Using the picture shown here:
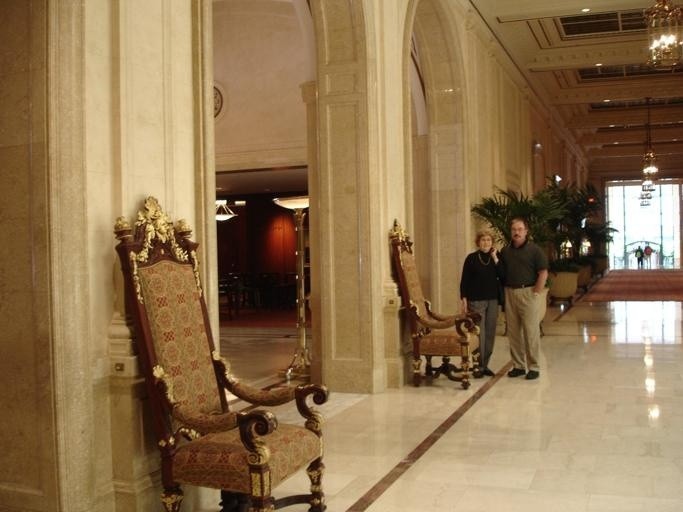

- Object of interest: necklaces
[478,254,490,265]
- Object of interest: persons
[496,215,548,379]
[635,245,644,271]
[459,229,506,377]
[643,241,652,270]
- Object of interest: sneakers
[484,367,495,376]
[508,368,525,377]
[525,371,539,379]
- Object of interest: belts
[508,284,535,289]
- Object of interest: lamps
[273,196,311,381]
[642,0,683,74]
[215,199,238,222]
[639,97,659,206]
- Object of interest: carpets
[583,269,683,302]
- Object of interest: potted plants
[530,174,619,307]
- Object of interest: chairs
[114,195,330,512]
[219,271,297,321]
[389,218,482,389]
